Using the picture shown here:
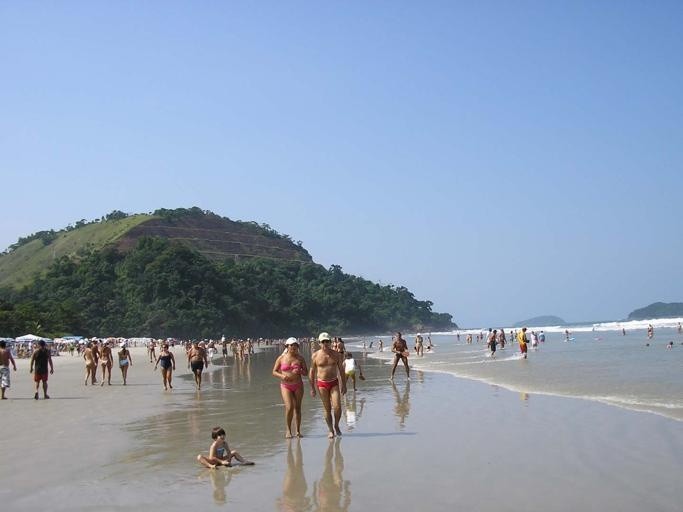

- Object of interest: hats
[318,332,331,342]
[285,336,299,346]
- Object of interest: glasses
[321,340,330,343]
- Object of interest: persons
[647,324,653,339]
[623,329,625,335]
[457,328,545,359]
[677,322,682,333]
[565,330,569,339]
[198,465,252,506]
[666,341,673,351]
[0,327,435,400]
[196,426,255,470]
[272,332,348,438]
[276,437,352,512]
